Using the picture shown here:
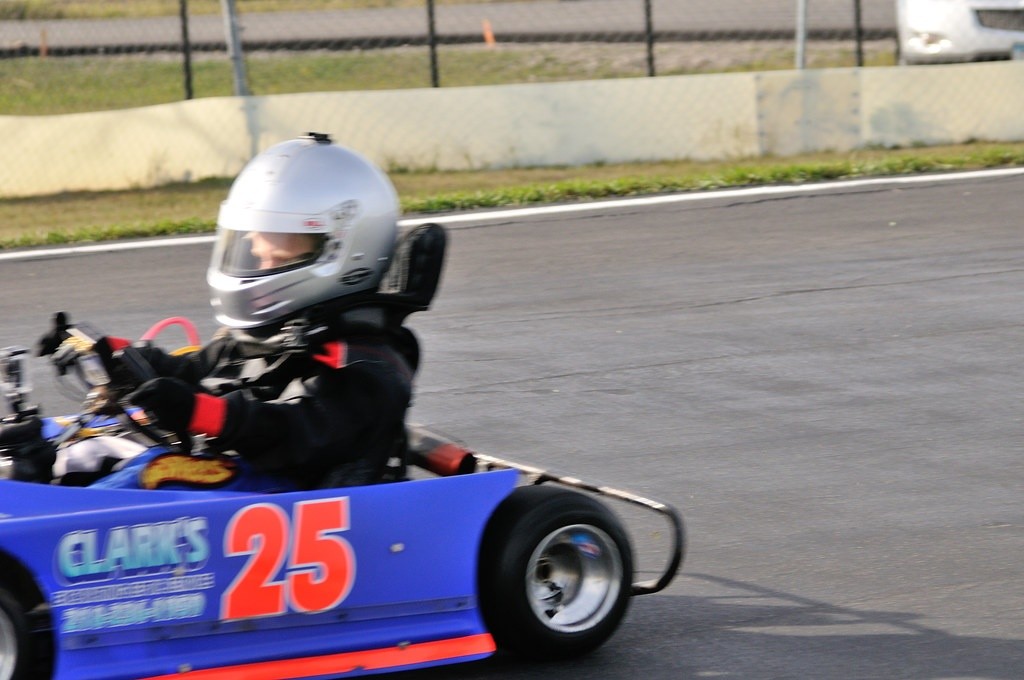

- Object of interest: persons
[51,135,414,496]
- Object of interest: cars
[895,1,1024,63]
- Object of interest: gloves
[40,314,136,377]
[121,377,247,459]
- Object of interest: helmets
[204,132,399,329]
